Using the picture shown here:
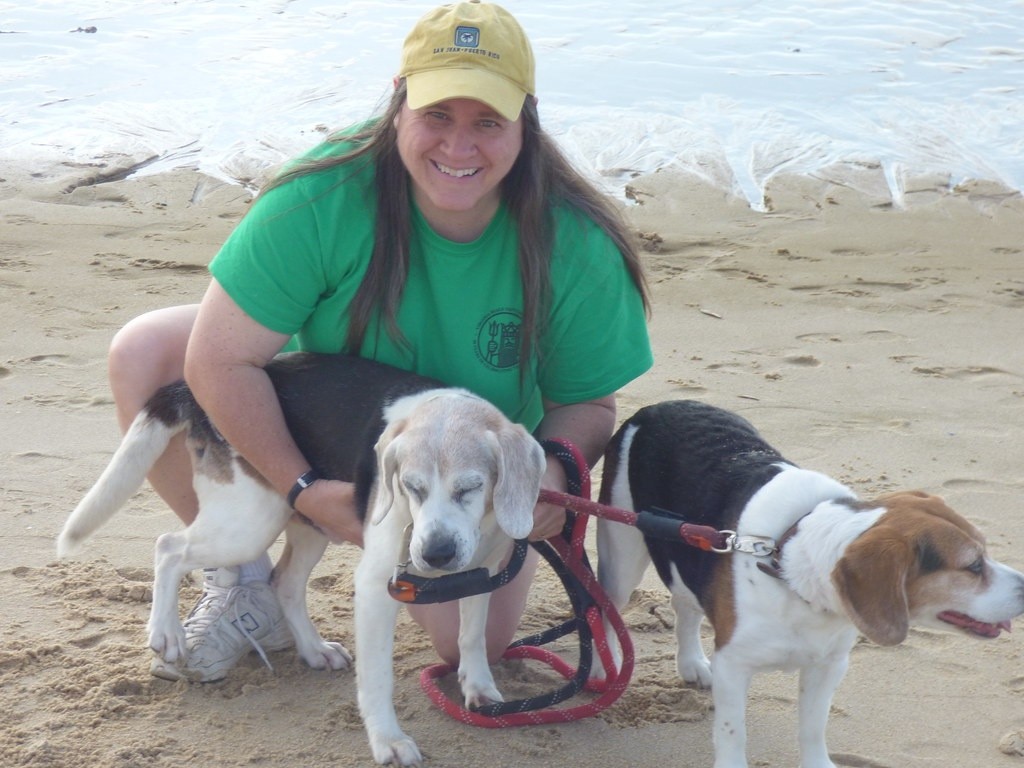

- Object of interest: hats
[396,0,535,122]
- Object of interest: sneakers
[149,563,295,683]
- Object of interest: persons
[109,0,653,678]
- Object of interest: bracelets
[287,468,319,510]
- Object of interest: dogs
[56,351,548,768]
[585,397,1024,768]
